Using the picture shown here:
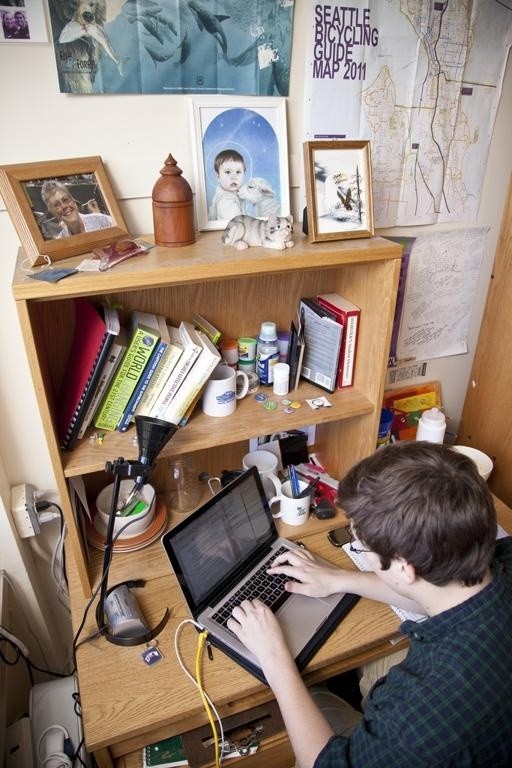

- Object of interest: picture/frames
[304,140,375,242]
[190,94,291,231]
[0,156,132,267]
[0,0,50,44]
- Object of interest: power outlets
[11,484,41,538]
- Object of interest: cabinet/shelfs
[12,220,403,599]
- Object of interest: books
[142,735,189,768]
[116,315,171,434]
[76,332,122,441]
[287,294,361,394]
[56,299,119,453]
[342,539,430,624]
[191,312,222,343]
[92,310,161,432]
[149,321,203,419]
[161,330,222,426]
[130,325,184,424]
[87,327,128,428]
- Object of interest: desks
[64,492,511,768]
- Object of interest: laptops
[159,464,354,673]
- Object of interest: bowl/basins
[96,473,157,529]
[451,445,493,483]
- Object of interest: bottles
[239,338,256,361]
[273,362,290,396]
[257,322,281,388]
[163,454,201,513]
[220,339,237,364]
[237,362,255,374]
[415,407,447,446]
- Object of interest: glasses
[349,534,373,555]
[52,196,71,213]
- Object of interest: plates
[85,504,167,553]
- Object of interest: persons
[206,150,246,221]
[41,181,116,240]
[227,441,512,768]
[14,12,30,38]
[3,12,18,38]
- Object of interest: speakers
[105,584,151,638]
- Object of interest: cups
[242,451,281,502]
[201,363,249,418]
[268,480,312,526]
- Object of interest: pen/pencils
[287,465,319,499]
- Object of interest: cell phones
[326,524,356,547]
